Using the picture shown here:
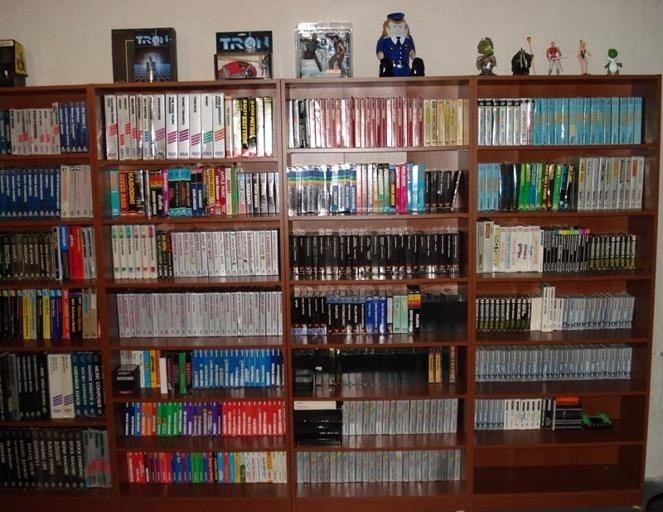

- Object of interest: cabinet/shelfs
[280,77,471,504]
[472,72,658,506]
[4,82,116,506]
[90,71,288,508]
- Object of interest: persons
[546,41,562,75]
[578,41,591,74]
[300,34,324,71]
[375,13,425,77]
[327,36,346,75]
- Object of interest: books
[117,350,288,484]
[477,95,645,210]
[1,225,101,345]
[291,348,460,445]
[475,346,633,429]
[110,223,283,337]
[476,221,637,334]
[286,97,466,215]
[296,449,461,484]
[292,228,463,336]
[1,350,114,489]
[102,93,281,217]
[1,100,95,219]
